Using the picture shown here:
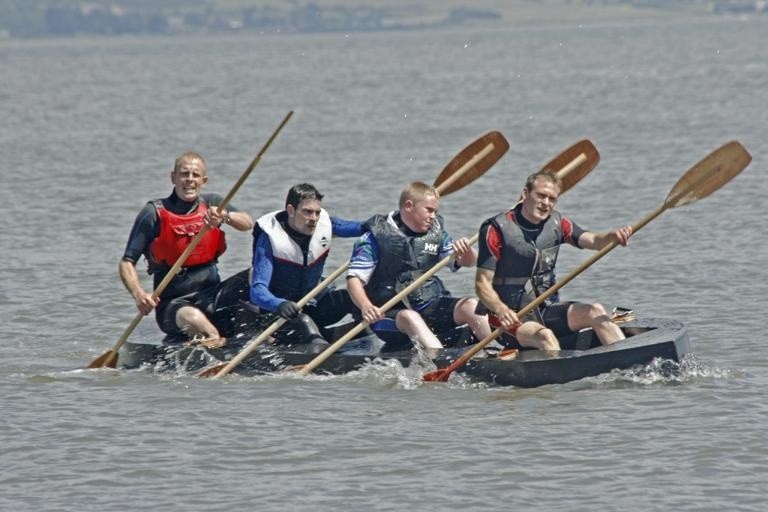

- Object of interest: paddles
[197,130,510,379]
[422,140,752,383]
[89,111,294,369]
[285,139,600,377]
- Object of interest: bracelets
[225,209,231,223]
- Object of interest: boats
[117,307,693,391]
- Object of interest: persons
[346,182,509,362]
[118,149,254,345]
[247,182,367,344]
[475,168,636,353]
